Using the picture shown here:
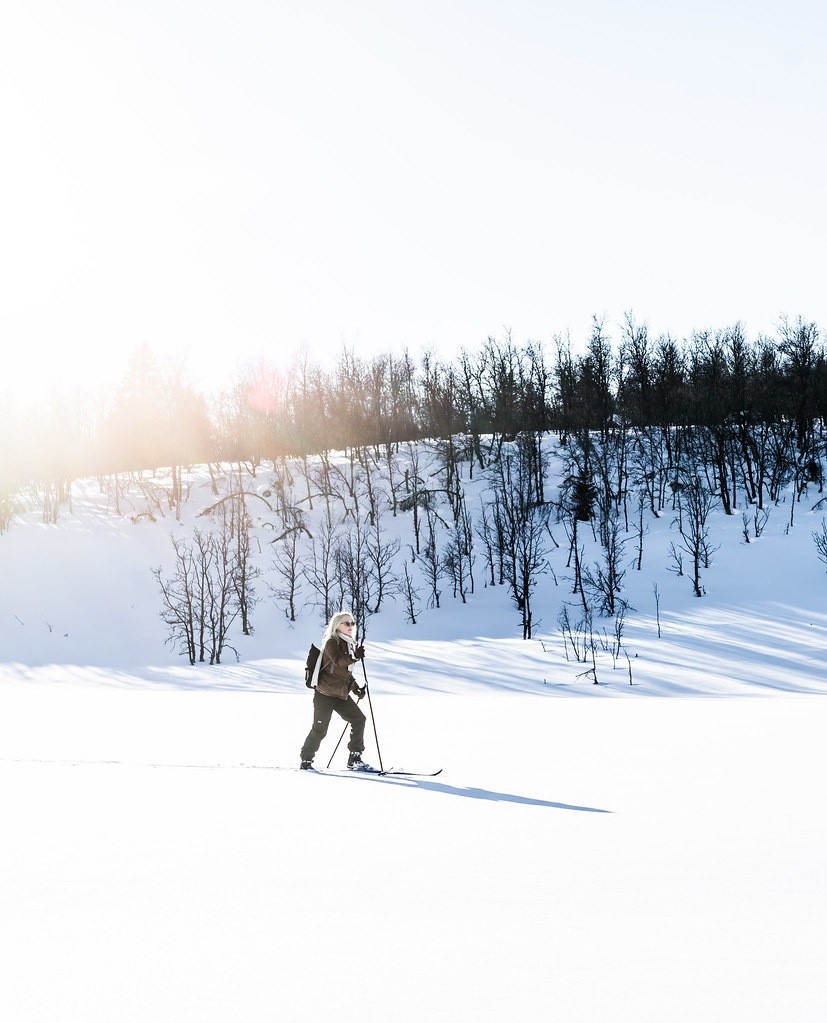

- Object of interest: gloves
[354,643,365,659]
[356,683,366,700]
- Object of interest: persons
[300,614,370,770]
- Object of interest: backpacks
[305,638,340,689]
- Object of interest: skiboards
[296,764,443,776]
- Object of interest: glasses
[339,621,355,626]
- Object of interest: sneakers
[300,760,315,772]
[347,752,369,768]
